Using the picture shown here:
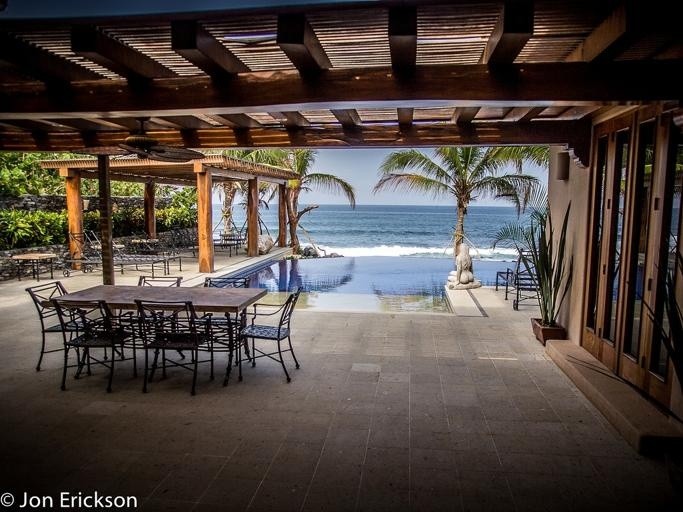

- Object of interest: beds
[11,252,57,282]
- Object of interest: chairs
[133,298,215,396]
[237,285,304,383]
[51,298,126,393]
[496,248,543,312]
[203,276,251,366]
[25,280,108,377]
[137,275,186,363]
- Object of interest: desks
[40,283,269,383]
